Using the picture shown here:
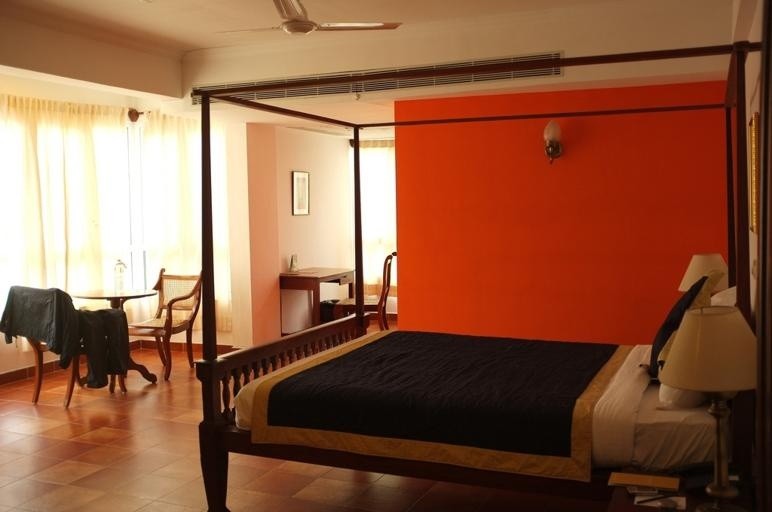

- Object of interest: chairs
[10,268,203,408]
[334,252,396,332]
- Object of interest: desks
[280,267,355,338]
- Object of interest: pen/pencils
[636,495,673,504]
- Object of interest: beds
[194,312,757,511]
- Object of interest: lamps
[543,120,562,163]
[678,253,728,292]
[657,305,759,497]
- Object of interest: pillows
[639,271,736,411]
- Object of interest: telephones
[290,254,298,273]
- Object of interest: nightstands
[608,465,757,511]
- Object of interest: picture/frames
[292,171,310,216]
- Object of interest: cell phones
[626,486,658,495]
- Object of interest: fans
[214,0,404,34]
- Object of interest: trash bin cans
[320,300,339,322]
